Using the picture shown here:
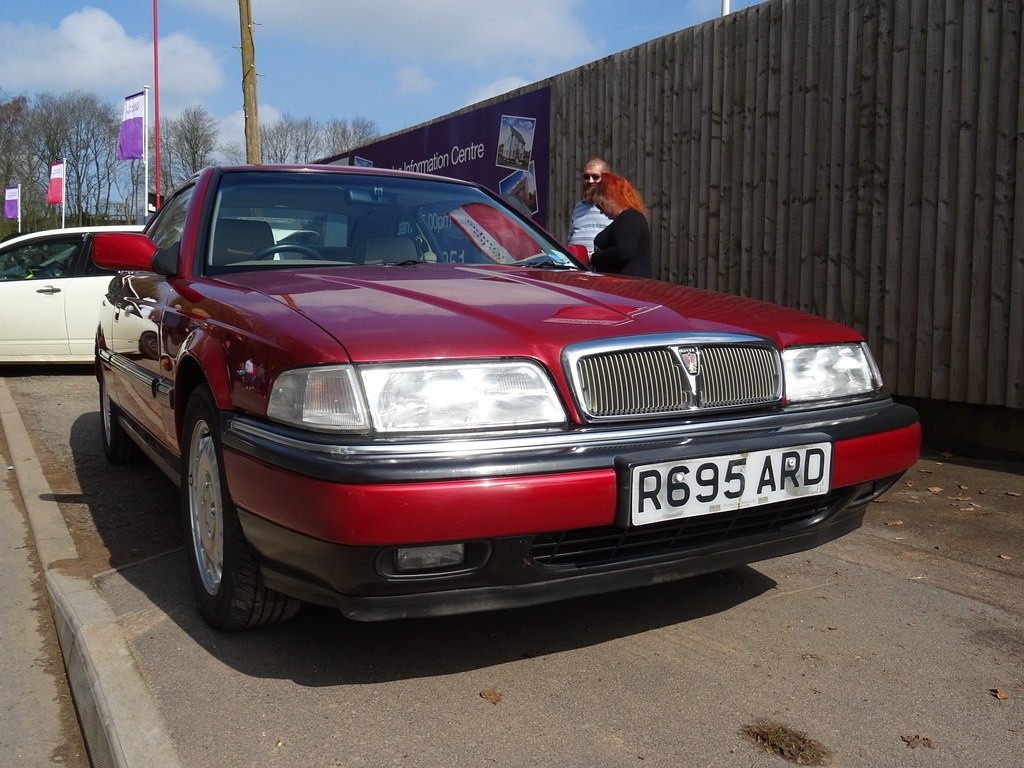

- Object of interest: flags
[117,91,145,160]
[47,162,63,204]
[5,188,18,219]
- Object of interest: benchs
[276,240,349,262]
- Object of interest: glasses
[594,200,604,209]
[583,174,600,180]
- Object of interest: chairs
[213,219,276,267]
[349,233,416,262]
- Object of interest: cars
[0,223,172,373]
[85,158,925,640]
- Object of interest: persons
[568,158,614,261]
[592,174,653,278]
